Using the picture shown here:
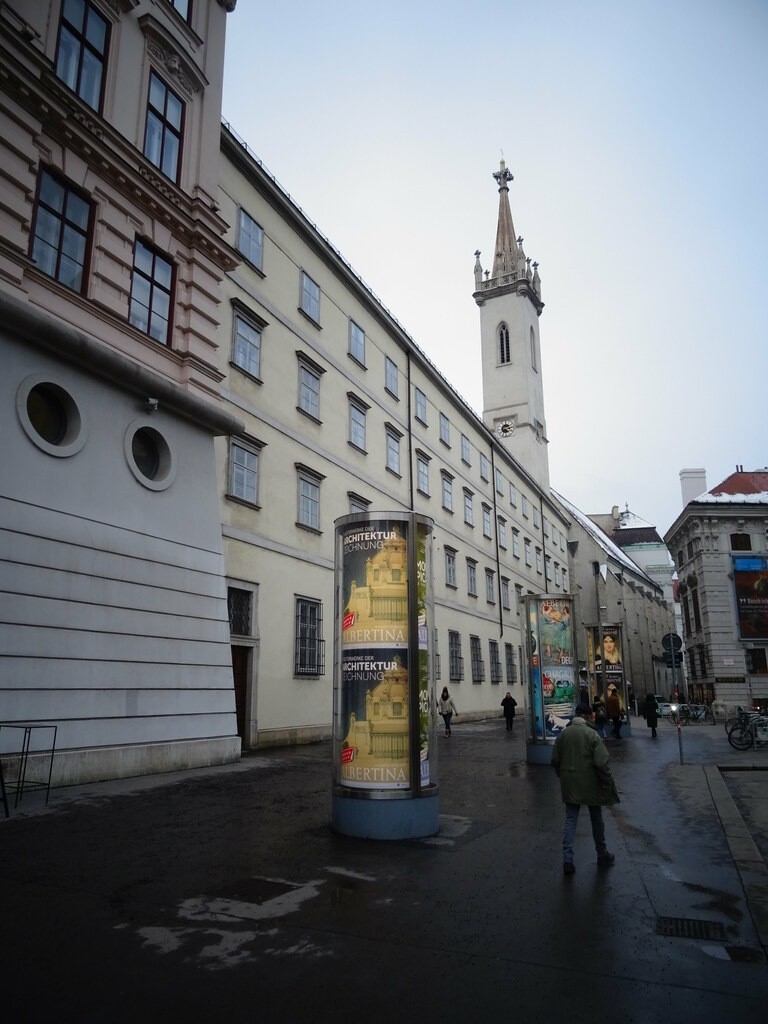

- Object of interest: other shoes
[652,730,657,737]
[617,733,622,739]
[563,863,575,874]
[598,853,615,865]
[611,731,617,737]
[449,728,451,735]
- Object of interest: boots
[444,729,448,738]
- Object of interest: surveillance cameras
[145,398,158,404]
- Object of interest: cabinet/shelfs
[0,724,57,808]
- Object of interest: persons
[592,696,608,740]
[501,692,518,732]
[596,633,621,664]
[551,704,619,874]
[438,687,458,738]
[607,689,624,740]
[642,693,659,738]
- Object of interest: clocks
[497,420,514,437]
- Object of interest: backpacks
[596,706,607,719]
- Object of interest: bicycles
[666,701,716,725]
[725,706,768,750]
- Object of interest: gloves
[456,714,458,716]
[438,713,441,715]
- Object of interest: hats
[575,703,593,714]
[443,687,448,692]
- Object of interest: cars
[656,702,710,718]
